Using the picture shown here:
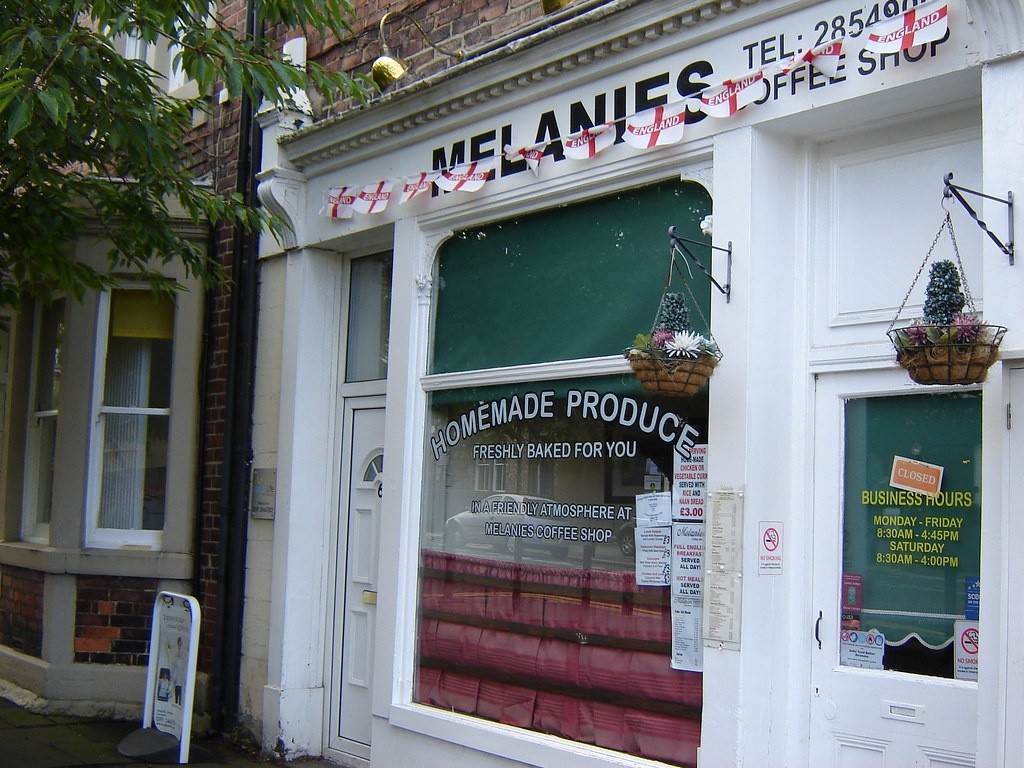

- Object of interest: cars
[444,491,570,555]
[841,536,975,612]
[617,516,638,557]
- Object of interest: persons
[166,637,187,707]
[158,669,169,702]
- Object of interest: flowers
[889,257,999,343]
[623,287,720,359]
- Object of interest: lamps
[371,10,466,94]
